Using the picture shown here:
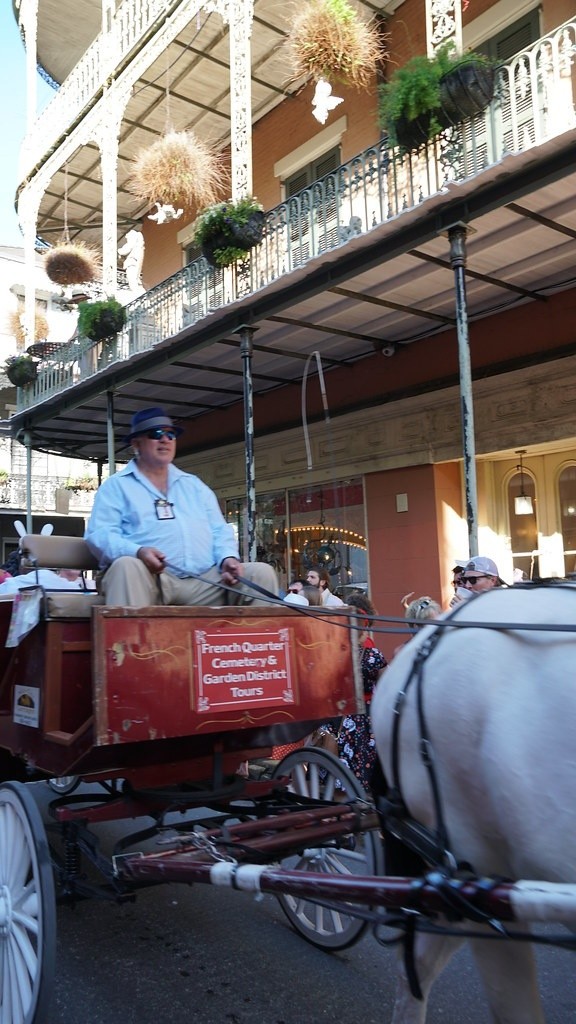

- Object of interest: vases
[196,211,263,269]
[4,362,38,387]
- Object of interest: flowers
[2,354,32,374]
[184,193,264,264]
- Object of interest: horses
[368,582,576,1024]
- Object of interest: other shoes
[342,836,356,851]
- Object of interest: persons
[402,556,510,638]
[273,565,387,852]
[0,548,98,595]
[86,408,280,607]
[118,230,144,299]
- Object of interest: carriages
[0,505,576,1024]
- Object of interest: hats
[122,407,186,443]
[455,556,499,577]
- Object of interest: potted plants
[374,43,498,150]
[75,293,127,342]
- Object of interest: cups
[456,587,473,599]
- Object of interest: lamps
[563,471,576,516]
[514,451,534,515]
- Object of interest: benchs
[18,534,107,727]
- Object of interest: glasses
[450,580,464,587]
[415,600,431,619]
[144,429,176,440]
[285,589,298,594]
[461,575,492,584]
